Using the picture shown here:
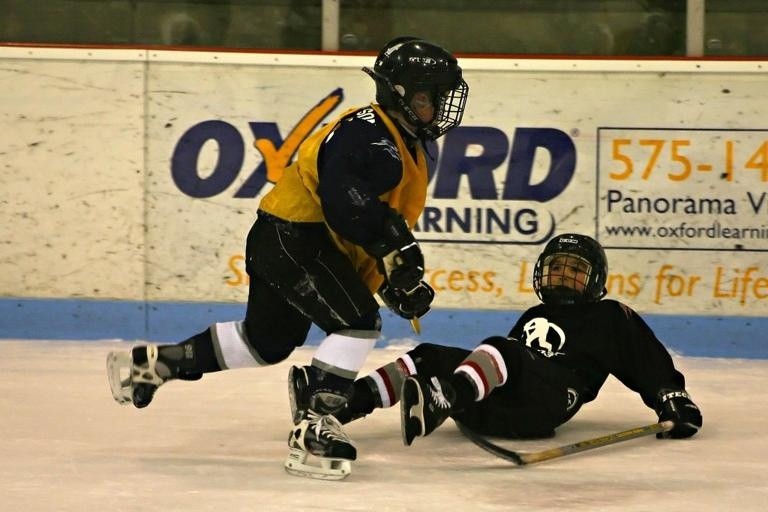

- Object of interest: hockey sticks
[455,419,676,465]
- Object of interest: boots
[287,362,374,462]
[129,338,203,409]
[407,370,472,443]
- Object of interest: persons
[131,34,470,461]
[287,233,704,448]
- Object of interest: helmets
[540,232,609,297]
[376,36,463,112]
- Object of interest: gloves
[366,219,436,320]
[656,391,703,439]
